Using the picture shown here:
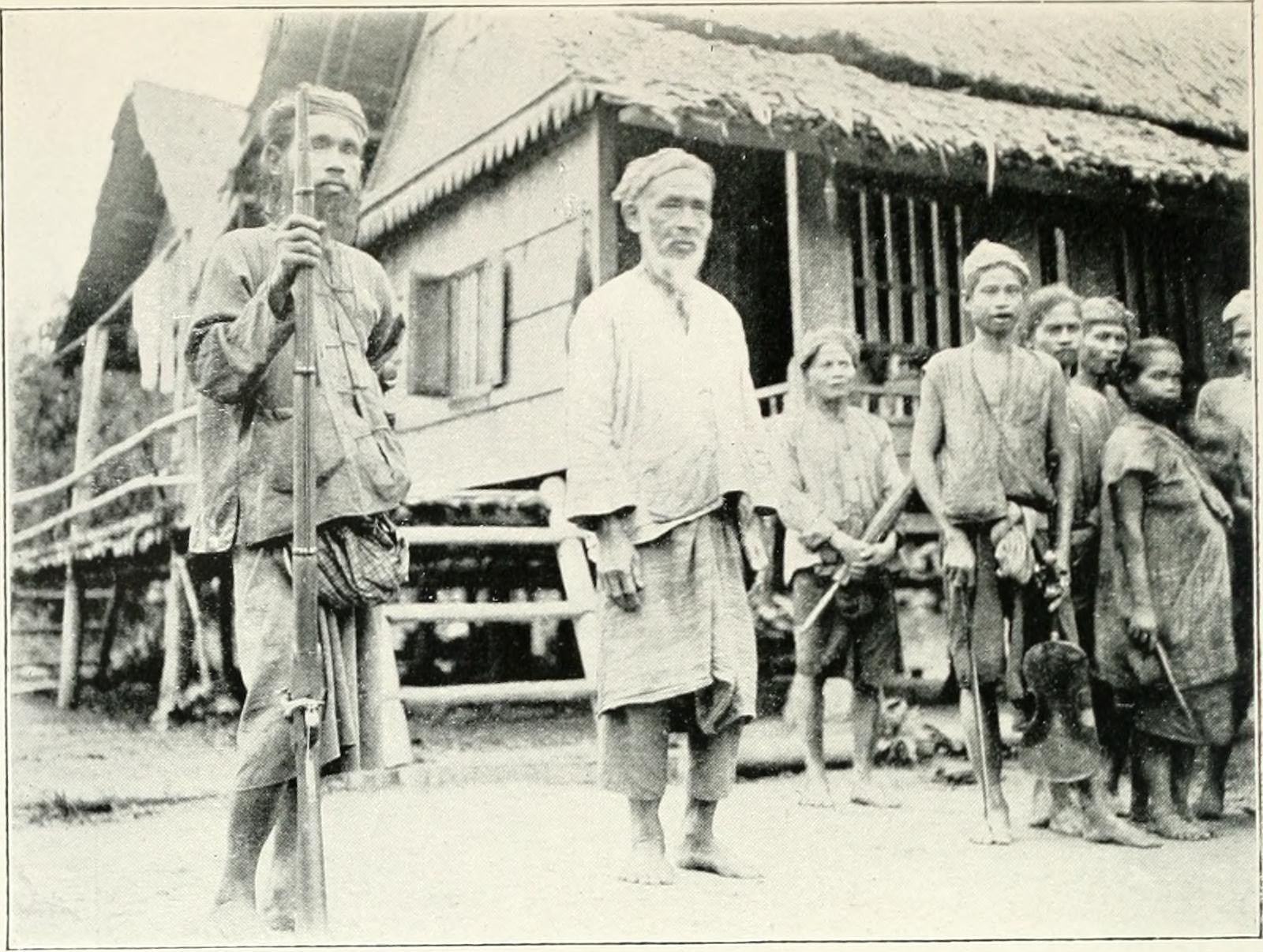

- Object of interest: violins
[1008,558,1107,786]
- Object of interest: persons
[560,147,772,887]
[180,84,414,943]
[902,235,1263,850]
[769,321,915,811]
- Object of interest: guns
[280,85,328,941]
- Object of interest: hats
[1081,295,1124,325]
[962,237,1033,295]
[1221,287,1253,323]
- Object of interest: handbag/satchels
[276,512,409,606]
